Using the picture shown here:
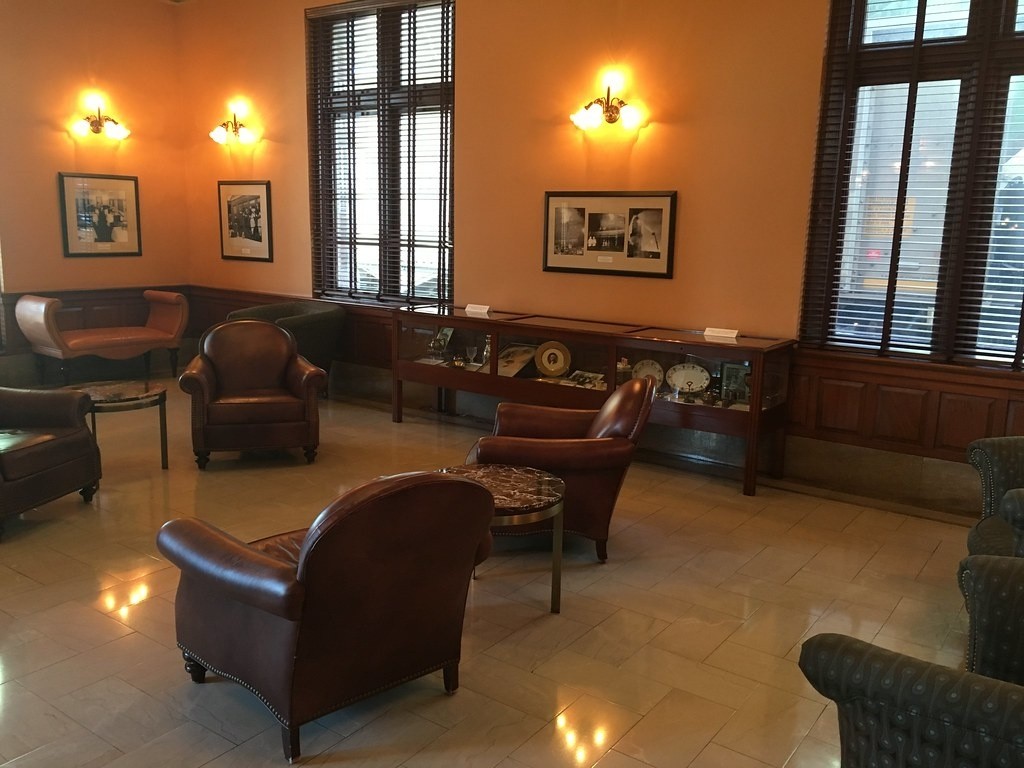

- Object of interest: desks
[435,459,563,615]
[57,381,170,472]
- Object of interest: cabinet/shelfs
[387,303,798,498]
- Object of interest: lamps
[210,98,255,146]
[572,68,645,132]
[69,94,132,141]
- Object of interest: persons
[549,355,557,364]
[588,236,596,249]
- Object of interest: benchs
[14,291,190,384]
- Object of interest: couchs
[157,470,494,764]
[178,320,328,472]
[227,301,345,400]
[468,373,657,561]
[797,435,1024,768]
[0,386,103,522]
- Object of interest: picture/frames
[57,171,143,258]
[542,189,677,279]
[216,181,274,263]
